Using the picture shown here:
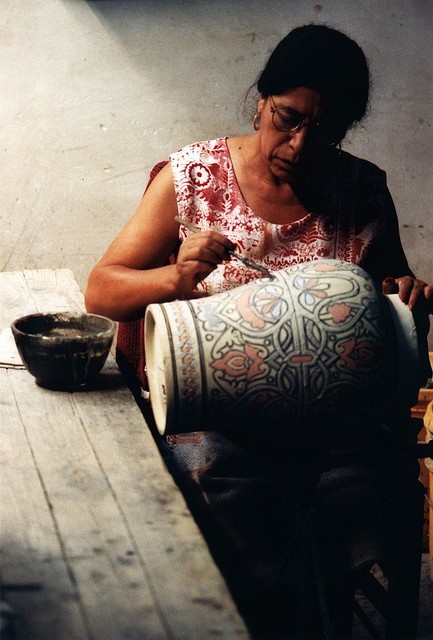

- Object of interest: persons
[84,19,433,640]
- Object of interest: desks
[0,268,249,640]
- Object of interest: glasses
[269,95,339,146]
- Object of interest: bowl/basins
[11,312,116,393]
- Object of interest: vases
[143,259,420,439]
